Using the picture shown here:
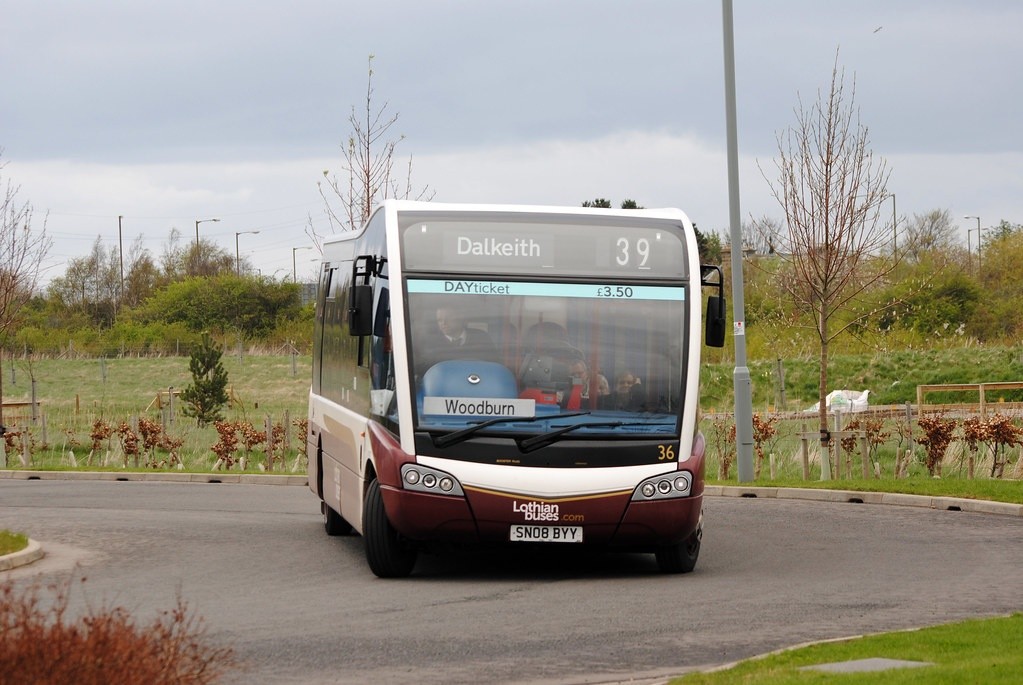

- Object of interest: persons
[551,356,646,412]
[411,299,503,378]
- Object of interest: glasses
[619,380,634,385]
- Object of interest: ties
[452,338,462,347]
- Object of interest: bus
[317,200,727,574]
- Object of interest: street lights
[967,228,988,270]
[293,245,313,282]
[964,215,982,273]
[195,219,221,276]
[235,230,260,277]
